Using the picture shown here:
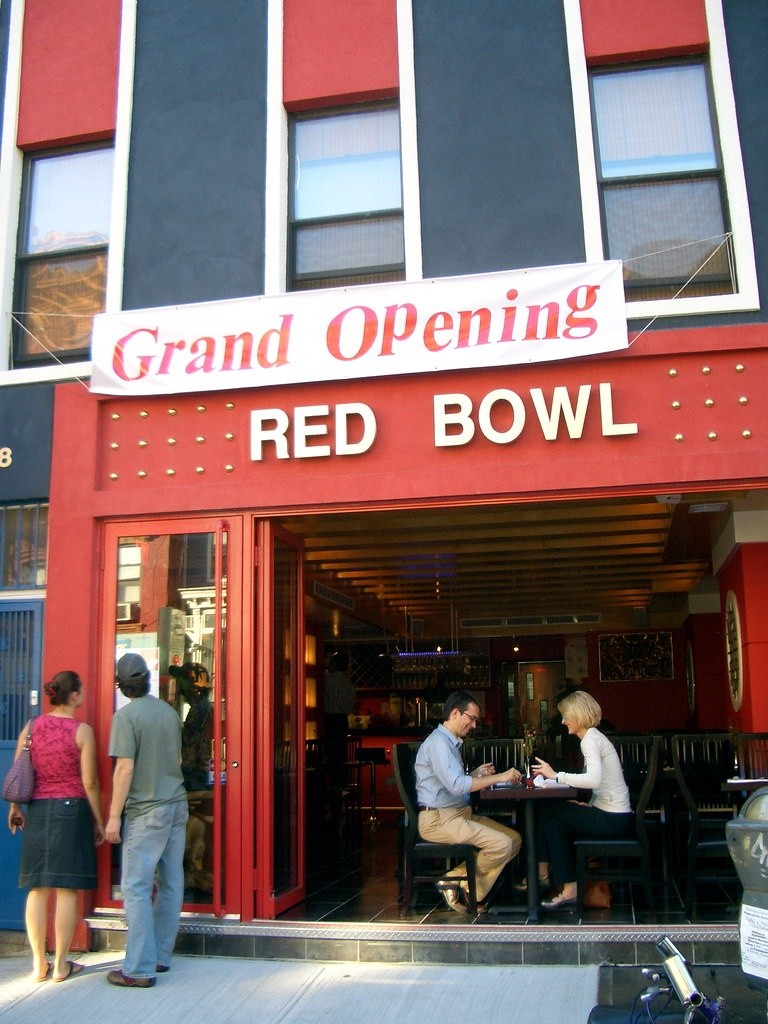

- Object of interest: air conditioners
[117,602,139,623]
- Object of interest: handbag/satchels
[583,856,611,908]
[2,716,35,803]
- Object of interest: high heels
[513,872,550,890]
[540,890,578,912]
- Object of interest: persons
[511,691,632,910]
[169,660,215,899]
[324,652,357,789]
[8,668,108,982]
[412,690,522,918]
[102,654,190,988]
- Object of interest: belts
[418,805,431,812]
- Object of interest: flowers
[523,722,539,758]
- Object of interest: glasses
[461,710,481,724]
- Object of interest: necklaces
[195,702,212,730]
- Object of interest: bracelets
[556,774,558,783]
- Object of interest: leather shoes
[458,887,488,913]
[436,874,469,913]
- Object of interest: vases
[525,755,535,789]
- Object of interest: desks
[478,778,577,923]
[720,780,768,797]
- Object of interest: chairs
[392,742,479,916]
[573,737,660,920]
[665,732,745,922]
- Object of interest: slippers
[34,960,52,981]
[54,961,84,982]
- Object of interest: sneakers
[107,968,156,988]
[156,964,170,972]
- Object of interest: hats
[169,662,212,692]
[117,653,149,682]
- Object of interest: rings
[540,765,542,767]
